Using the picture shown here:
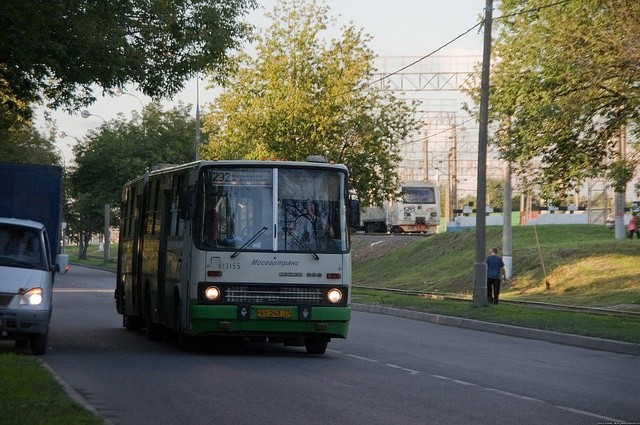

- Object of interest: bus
[114,156,360,354]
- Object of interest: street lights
[113,87,149,174]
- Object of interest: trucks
[0,162,68,354]
[361,181,441,234]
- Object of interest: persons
[98,235,104,251]
[486,248,506,304]
[293,198,335,241]
[628,211,639,240]
[6,240,34,263]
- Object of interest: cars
[606,201,640,229]
